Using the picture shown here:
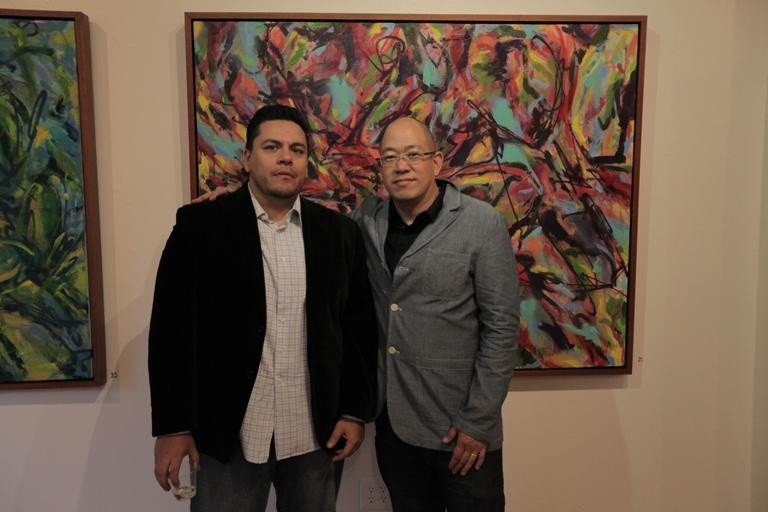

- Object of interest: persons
[146,102,383,512]
[188,116,526,512]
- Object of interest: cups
[167,457,198,500]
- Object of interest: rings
[470,453,479,458]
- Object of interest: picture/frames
[0,7,105,390]
[184,14,648,377]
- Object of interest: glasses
[374,151,435,167]
